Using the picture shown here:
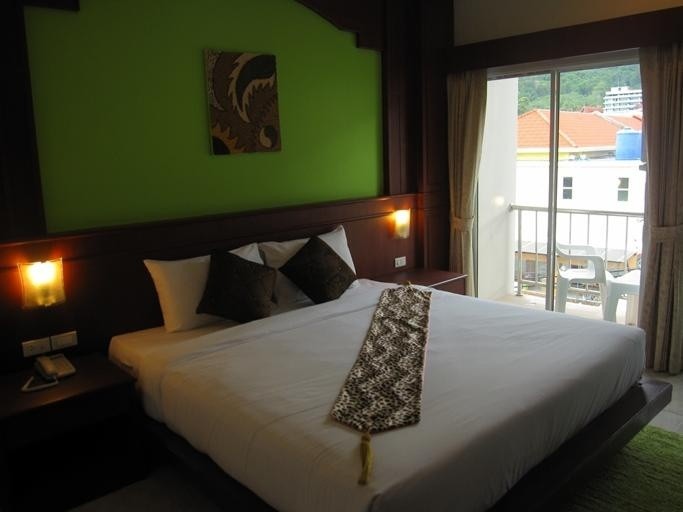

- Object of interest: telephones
[32,353,76,383]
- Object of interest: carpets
[574,422,683,512]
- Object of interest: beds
[108,279,671,511]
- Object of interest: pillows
[278,237,357,305]
[142,242,264,333]
[194,248,278,321]
[260,226,356,305]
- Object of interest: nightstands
[382,266,467,295]
[2,355,136,510]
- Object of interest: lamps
[20,258,66,310]
[395,210,410,241]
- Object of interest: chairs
[553,243,614,321]
[608,269,639,323]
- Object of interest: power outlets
[21,337,50,355]
